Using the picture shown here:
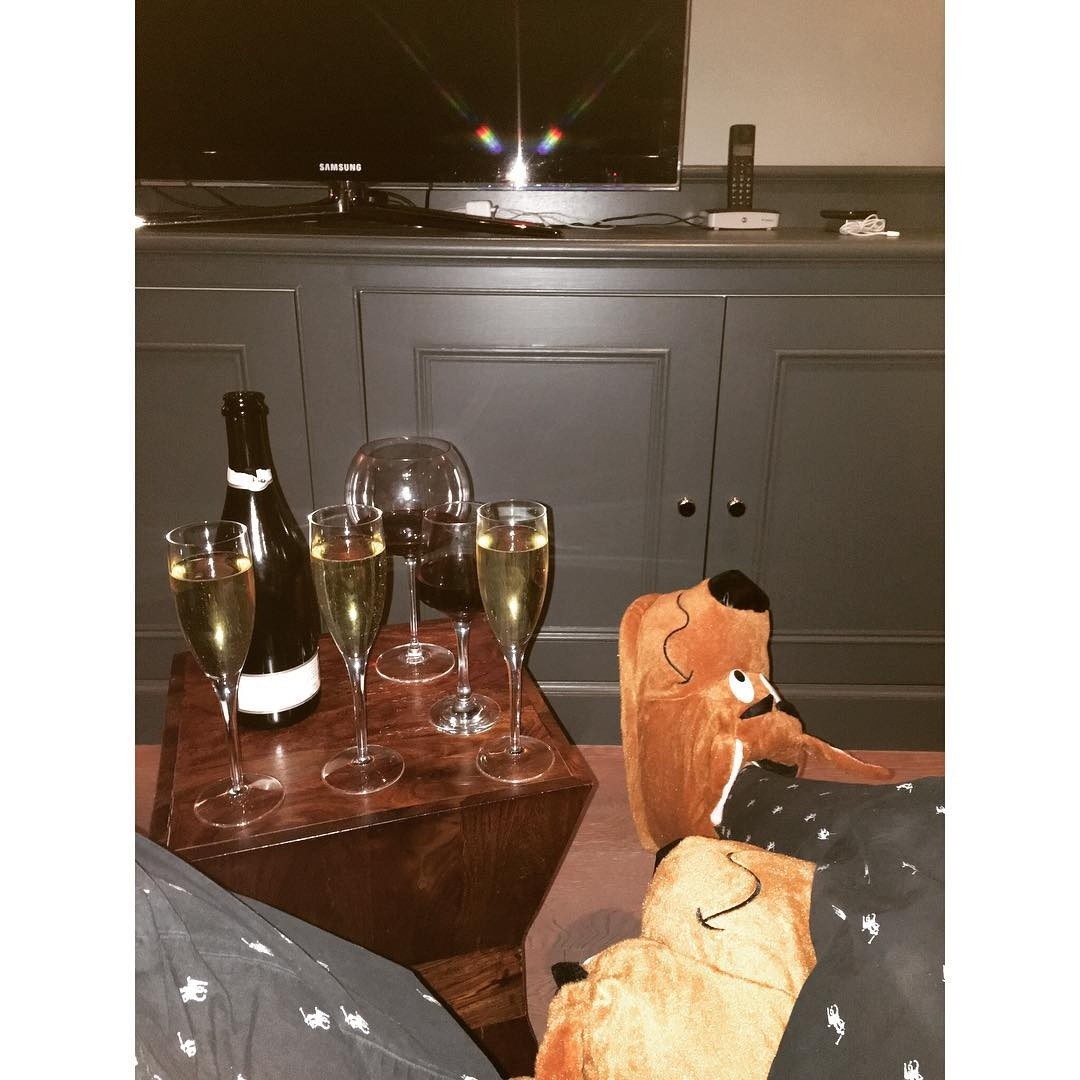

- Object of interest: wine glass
[475,500,556,782]
[164,520,283,827]
[305,504,404,795]
[414,501,502,736]
[344,435,471,683]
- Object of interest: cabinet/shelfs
[136,236,333,680]
[333,242,944,751]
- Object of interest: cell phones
[821,210,877,220]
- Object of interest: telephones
[700,123,783,234]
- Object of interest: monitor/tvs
[135,0,690,192]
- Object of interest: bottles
[211,391,321,731]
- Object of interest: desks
[154,618,596,1044]
